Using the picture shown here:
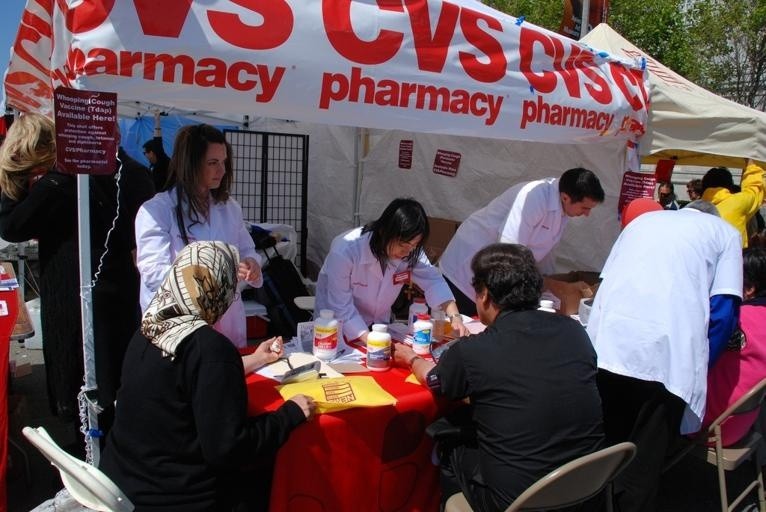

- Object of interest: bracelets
[406,354,424,373]
[448,313,464,324]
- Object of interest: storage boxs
[414,214,466,266]
[539,266,602,319]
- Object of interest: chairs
[21,422,139,512]
[705,376,765,512]
[441,443,637,511]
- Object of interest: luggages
[253,231,314,338]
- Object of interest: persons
[438,167,605,316]
[390,243,609,511]
[315,195,472,346]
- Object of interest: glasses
[142,152,146,156]
[231,292,240,303]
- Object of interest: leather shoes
[53,399,74,422]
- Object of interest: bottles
[431,307,445,342]
[407,296,429,335]
[365,322,391,371]
[312,308,339,361]
[537,299,556,313]
[412,314,433,355]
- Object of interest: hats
[687,180,702,192]
[621,199,665,228]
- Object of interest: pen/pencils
[329,349,346,362]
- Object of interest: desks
[233,310,497,512]
